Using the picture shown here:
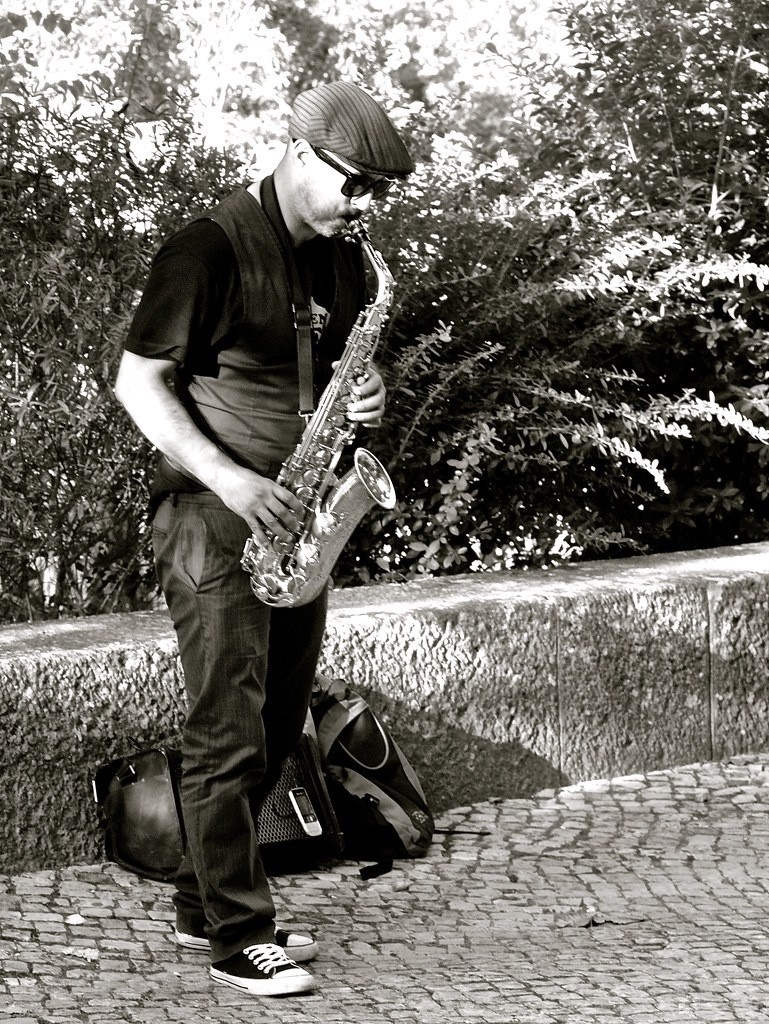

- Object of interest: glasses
[291,137,395,200]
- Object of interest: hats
[288,81,414,181]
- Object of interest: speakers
[255,733,346,875]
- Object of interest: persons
[110,80,417,997]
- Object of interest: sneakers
[175,925,318,961]
[208,943,316,996]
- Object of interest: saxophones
[242,216,398,608]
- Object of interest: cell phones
[287,786,323,836]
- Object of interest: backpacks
[309,671,492,882]
